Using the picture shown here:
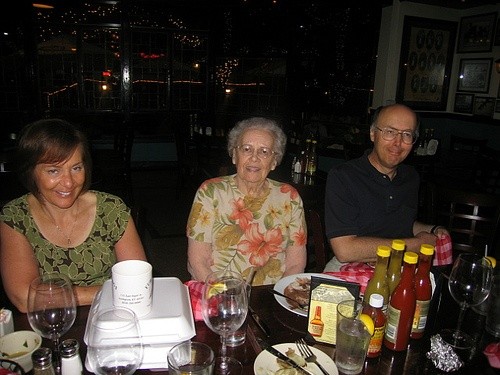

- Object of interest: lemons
[482,257,496,268]
[208,283,228,299]
[359,315,374,334]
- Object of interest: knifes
[255,337,312,375]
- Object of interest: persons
[324,103,452,274]
[0,119,148,315]
[186,117,307,288]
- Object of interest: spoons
[271,289,308,309]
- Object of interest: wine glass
[27,273,76,375]
[201,270,249,375]
[436,253,492,350]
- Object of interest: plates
[273,272,346,317]
[254,344,339,375]
[401,266,436,298]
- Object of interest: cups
[486,275,500,338]
[213,278,251,347]
[471,257,500,316]
[335,300,377,375]
[86,260,215,375]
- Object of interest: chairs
[445,190,500,255]
[93,119,134,195]
[193,134,227,168]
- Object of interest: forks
[295,338,330,375]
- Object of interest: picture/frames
[455,93,475,114]
[457,12,498,54]
[395,15,458,111]
[457,58,495,93]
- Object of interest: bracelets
[431,225,442,235]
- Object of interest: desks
[0,284,500,375]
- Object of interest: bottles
[383,251,419,353]
[289,127,317,177]
[363,246,391,315]
[309,306,324,337]
[410,243,435,339]
[362,293,386,358]
[414,128,434,150]
[387,240,406,303]
[31,339,84,375]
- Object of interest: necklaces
[42,196,80,245]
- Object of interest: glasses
[234,144,276,158]
[375,126,417,145]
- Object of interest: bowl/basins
[82,277,197,373]
[0,330,42,373]
